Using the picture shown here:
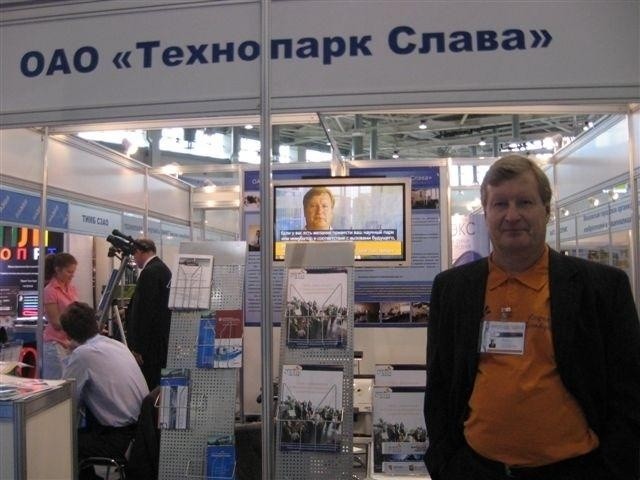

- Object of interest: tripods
[108,299,128,348]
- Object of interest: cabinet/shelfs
[0,373,77,479]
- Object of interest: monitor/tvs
[272,176,411,268]
[16,290,45,322]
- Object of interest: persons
[126,238,173,383]
[42,253,108,382]
[421,157,640,480]
[286,297,348,347]
[281,397,340,443]
[298,187,339,240]
[63,301,147,480]
[374,418,428,442]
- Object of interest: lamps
[549,186,619,222]
[419,120,427,130]
[583,122,589,131]
[479,137,486,145]
[122,139,136,155]
[204,179,218,194]
[393,151,399,159]
